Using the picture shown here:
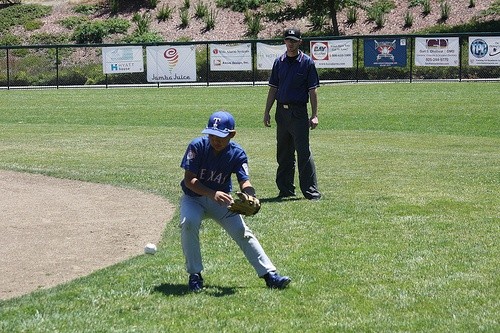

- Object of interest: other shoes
[306,192,321,200]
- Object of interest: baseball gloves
[221,190,261,220]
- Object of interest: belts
[277,103,307,110]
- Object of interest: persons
[263,29,322,199]
[179,111,291,290]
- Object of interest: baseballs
[145,243,157,255]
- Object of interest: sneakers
[189,273,204,292]
[264,270,293,290]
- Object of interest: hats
[201,110,236,138]
[283,29,301,42]
